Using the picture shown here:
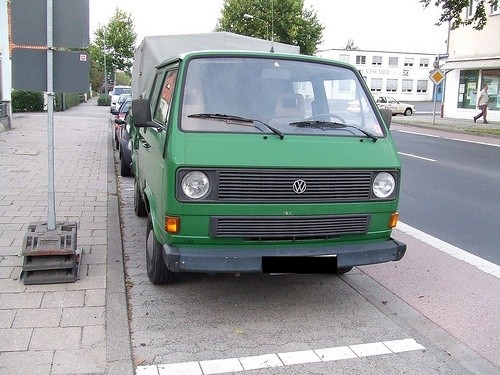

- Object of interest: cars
[115,107,132,177]
[347,96,416,116]
[114,96,132,150]
[113,93,131,114]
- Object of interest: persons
[473,84,490,124]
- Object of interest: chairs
[182,88,205,114]
[276,94,306,119]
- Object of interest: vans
[109,86,131,113]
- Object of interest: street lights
[104,17,134,105]
[244,14,268,40]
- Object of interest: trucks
[122,31,407,287]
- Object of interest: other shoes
[474,117,476,123]
[483,121,490,124]
